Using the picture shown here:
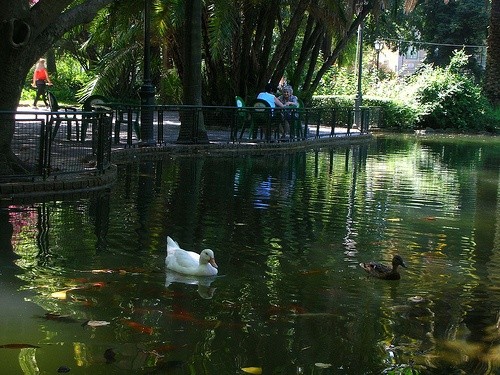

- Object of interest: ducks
[359,255,407,281]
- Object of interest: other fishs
[0,217,449,375]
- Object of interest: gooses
[165,236,219,276]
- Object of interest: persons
[276,85,297,139]
[33,58,51,109]
[257,85,283,142]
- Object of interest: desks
[94,102,144,146]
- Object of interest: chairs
[247,99,279,144]
[235,95,252,139]
[114,95,141,143]
[81,95,114,144]
[287,99,304,139]
[47,92,80,143]
